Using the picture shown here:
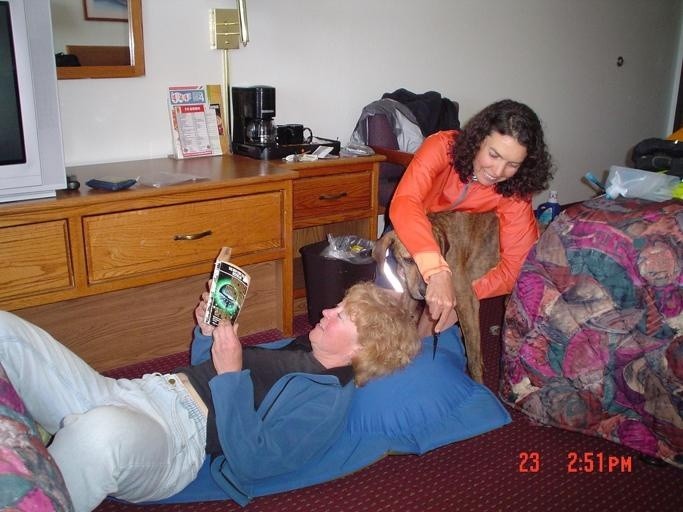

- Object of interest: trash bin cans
[299,235,378,327]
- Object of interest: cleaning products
[537,190,561,226]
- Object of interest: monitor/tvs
[0,0,67,203]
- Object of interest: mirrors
[50,0,145,80]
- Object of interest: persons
[0,280,423,512]
[379,99,556,342]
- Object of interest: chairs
[366,115,414,214]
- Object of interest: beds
[499,194,683,470]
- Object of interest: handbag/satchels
[632,139,682,180]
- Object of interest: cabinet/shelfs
[265,155,387,317]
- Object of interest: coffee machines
[231,84,299,160]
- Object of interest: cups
[276,124,312,145]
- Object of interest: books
[204,247,252,329]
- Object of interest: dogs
[370,209,549,386]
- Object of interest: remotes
[64,173,80,190]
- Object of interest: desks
[0,154,299,373]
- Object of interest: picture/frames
[83,0,128,22]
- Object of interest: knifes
[432,318,440,361]
